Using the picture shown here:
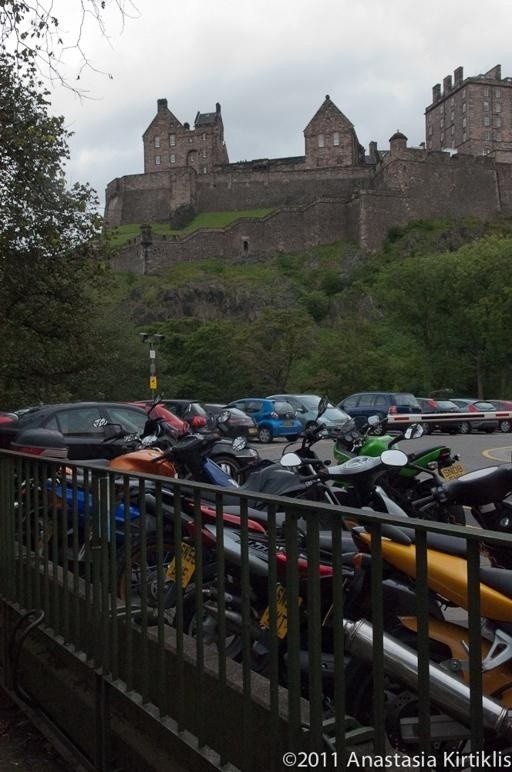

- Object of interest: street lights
[140,332,166,401]
